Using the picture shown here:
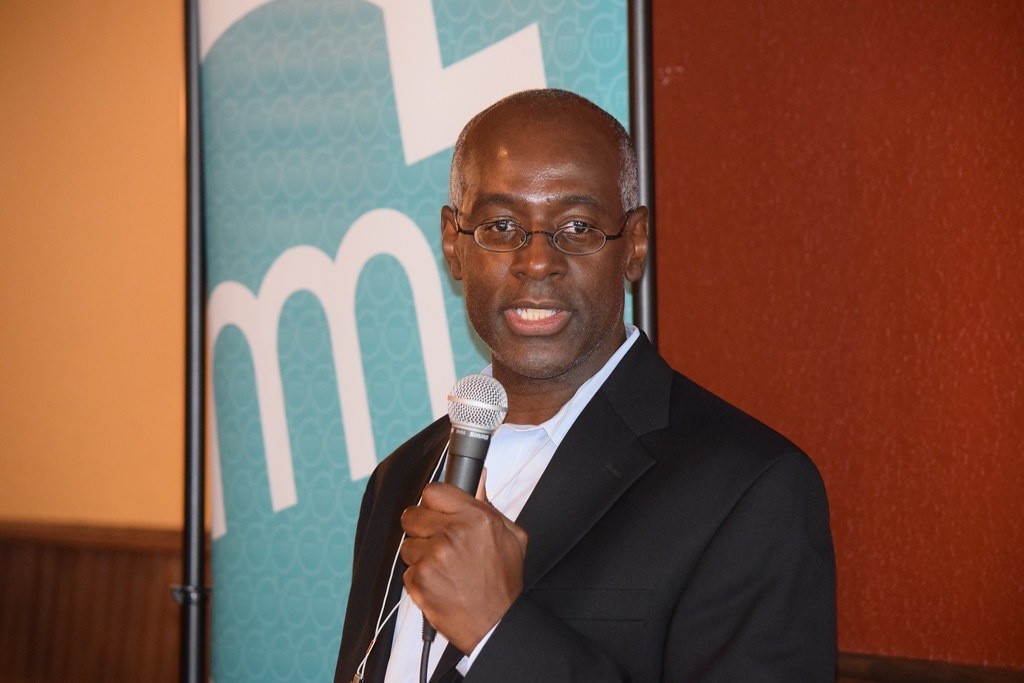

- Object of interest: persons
[333,88,837,683]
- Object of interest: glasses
[453,206,634,255]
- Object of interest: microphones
[421,373,509,641]
